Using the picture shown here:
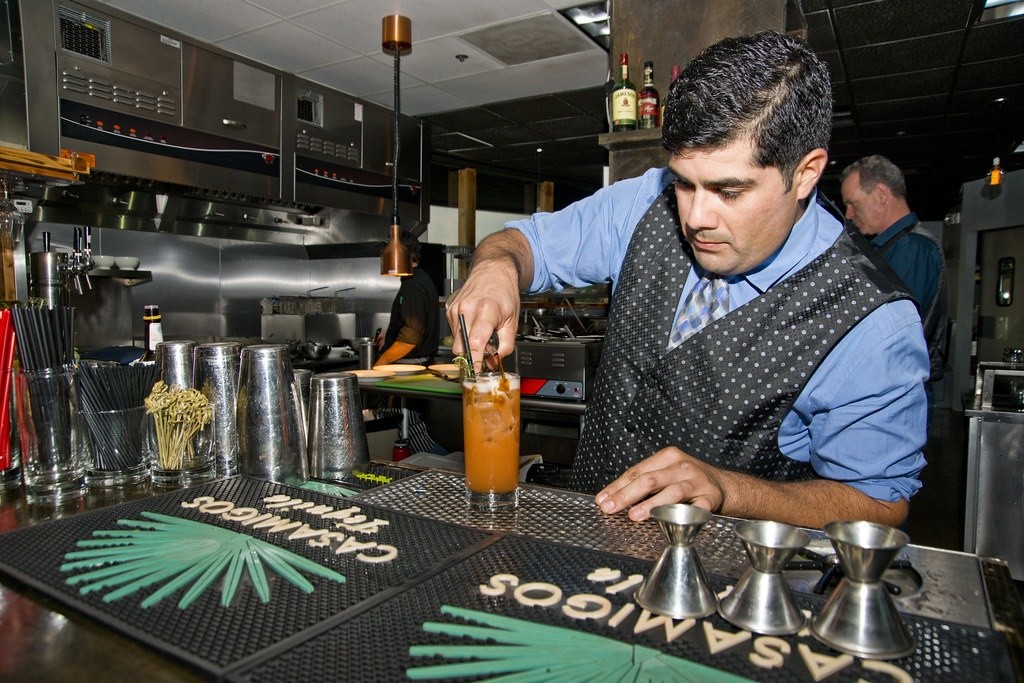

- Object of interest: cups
[462,370,520,512]
[17,368,89,505]
[0,369,24,493]
[146,407,216,490]
[811,521,920,659]
[632,502,723,617]
[71,406,153,488]
[718,519,811,636]
[157,335,371,480]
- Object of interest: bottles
[660,65,682,126]
[612,52,637,132]
[635,60,660,129]
[140,304,165,363]
[392,440,408,459]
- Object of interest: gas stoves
[502,296,605,402]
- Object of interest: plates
[428,364,472,375]
[341,369,396,382]
[372,364,427,375]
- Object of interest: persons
[374,231,437,456]
[839,154,948,437]
[444,31,930,530]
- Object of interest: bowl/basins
[114,256,140,270]
[299,341,332,360]
[91,255,115,270]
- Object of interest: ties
[665,270,730,354]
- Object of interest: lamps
[382,14,415,277]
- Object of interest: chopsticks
[0,303,75,474]
[67,363,163,469]
